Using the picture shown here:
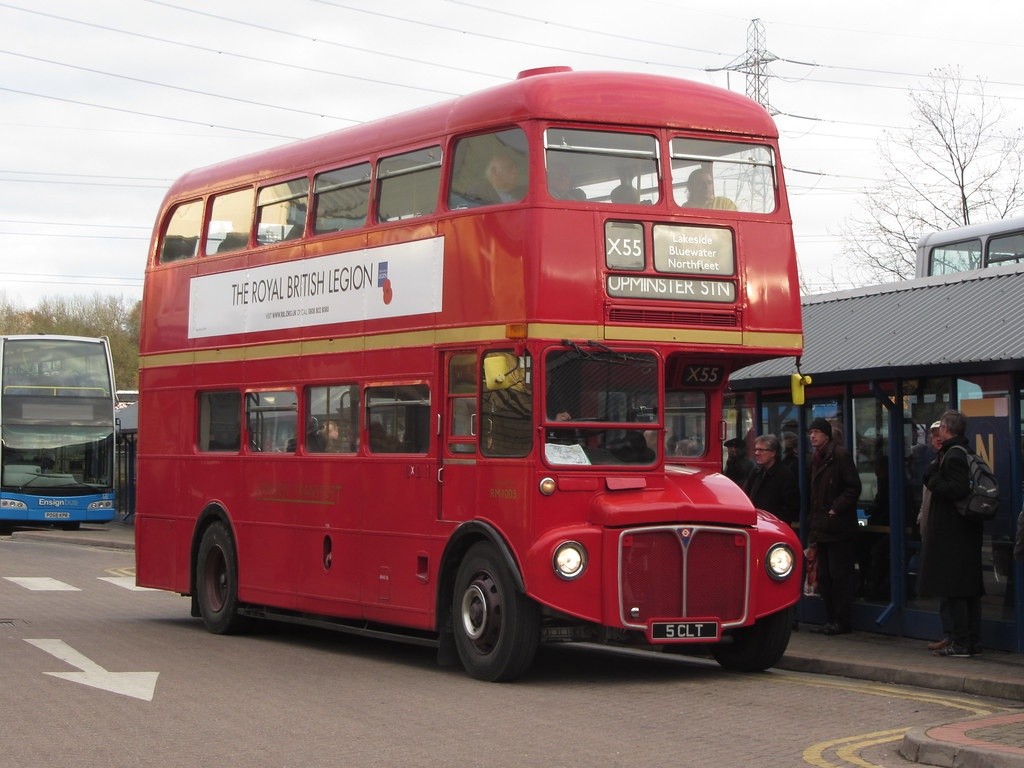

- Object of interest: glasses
[754,448,773,452]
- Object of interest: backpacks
[938,445,1002,520]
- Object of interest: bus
[0,334,122,530]
[133,65,811,681]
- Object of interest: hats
[929,421,942,430]
[807,416,833,439]
[723,437,746,448]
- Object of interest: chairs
[205,423,406,453]
[162,222,307,266]
[4,365,98,397]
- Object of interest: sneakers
[811,618,852,636]
[943,641,986,657]
[927,637,952,650]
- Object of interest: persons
[287,417,325,452]
[547,161,587,201]
[322,423,351,453]
[643,428,699,457]
[473,155,517,203]
[610,167,640,203]
[681,169,738,211]
[909,410,986,658]
[529,363,579,439]
[724,418,862,635]
